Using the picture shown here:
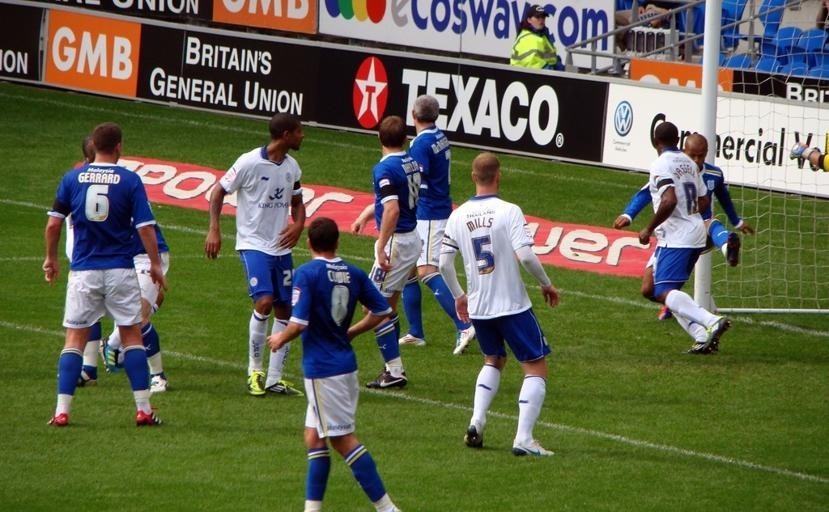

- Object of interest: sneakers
[454,325,477,356]
[705,316,732,352]
[511,436,554,458]
[688,329,711,353]
[374,370,407,389]
[725,234,741,266]
[658,303,672,321]
[77,370,99,385]
[247,370,267,397]
[151,375,169,393]
[136,409,165,426]
[464,418,484,449]
[98,335,121,374]
[48,412,69,426]
[263,381,305,398]
[366,368,388,388]
[398,333,428,346]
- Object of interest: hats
[525,5,549,18]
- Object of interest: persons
[615,0,681,56]
[509,4,566,72]
[42,122,168,427]
[351,116,422,389]
[790,142,829,172]
[638,121,732,356]
[397,93,477,354]
[611,133,755,321]
[204,112,308,398]
[440,152,560,458]
[265,218,402,512]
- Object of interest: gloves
[789,141,815,161]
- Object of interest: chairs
[738,0,786,45]
[727,54,751,70]
[783,28,828,67]
[809,63,829,78]
[698,53,725,68]
[811,43,829,67]
[755,55,779,73]
[680,0,747,48]
[758,27,799,64]
[781,61,809,76]
